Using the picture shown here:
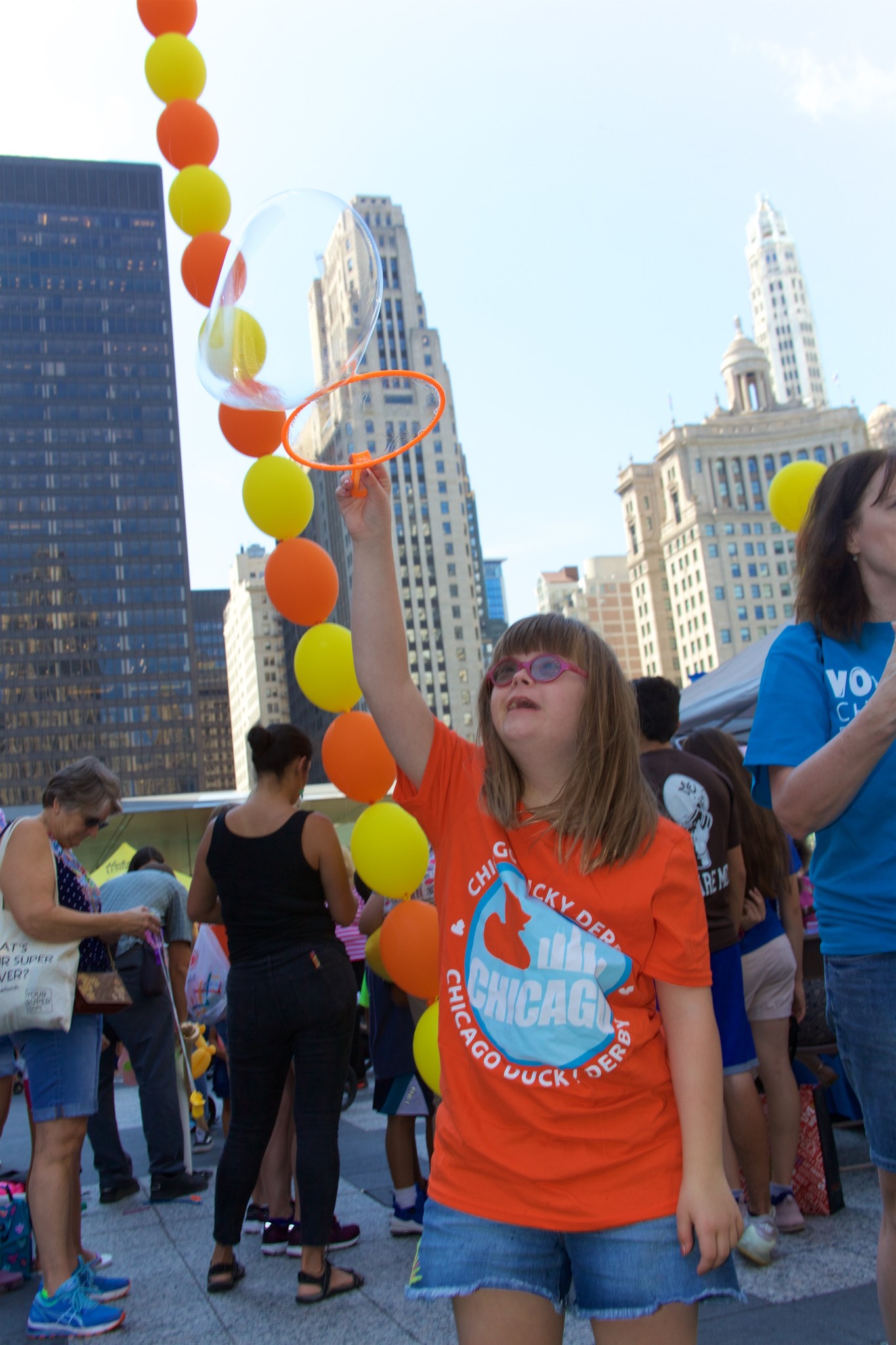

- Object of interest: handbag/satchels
[0,816,82,1036]
[186,924,229,1024]
[0,1184,33,1280]
[760,1015,844,1213]
[75,946,134,1011]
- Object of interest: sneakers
[191,1128,214,1154]
[26,1275,126,1340]
[37,1254,131,1300]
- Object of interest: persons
[352,841,436,1234]
[186,724,366,1303]
[794,1039,839,1089]
[0,803,13,1136]
[744,447,896,1344]
[333,454,744,1345]
[0,756,161,1335]
[85,843,227,1205]
[629,678,780,1265]
[681,730,809,1233]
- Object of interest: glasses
[86,816,108,832]
[486,654,589,694]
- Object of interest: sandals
[204,1253,245,1291]
[296,1260,364,1304]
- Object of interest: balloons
[265,537,340,627]
[157,101,218,169]
[380,900,439,1001]
[219,379,285,458]
[200,307,267,379]
[145,32,208,102]
[294,621,362,713]
[767,460,829,534]
[137,0,198,35]
[321,711,397,803]
[352,801,429,900]
[413,1000,443,1098]
[182,233,248,307]
[169,164,231,233]
[240,455,316,536]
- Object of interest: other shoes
[261,1218,289,1253]
[388,1189,426,1232]
[99,1177,139,1204]
[150,1176,208,1203]
[242,1206,268,1234]
[739,1206,777,1266]
[286,1219,361,1257]
[91,1254,113,1269]
[771,1190,806,1231]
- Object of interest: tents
[673,617,802,740]
